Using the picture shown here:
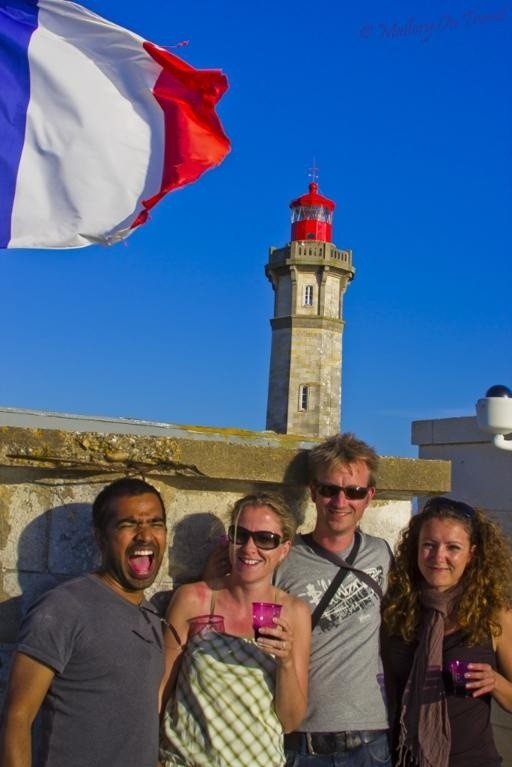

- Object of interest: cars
[447,661,477,700]
[252,600,283,644]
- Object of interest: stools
[282,731,381,755]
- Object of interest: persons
[264,431,399,764]
[2,475,173,767]
[369,496,511,764]
[149,491,315,766]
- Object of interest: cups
[317,484,370,499]
[228,526,280,550]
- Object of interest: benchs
[0,1,236,254]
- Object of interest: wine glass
[281,641,285,648]
